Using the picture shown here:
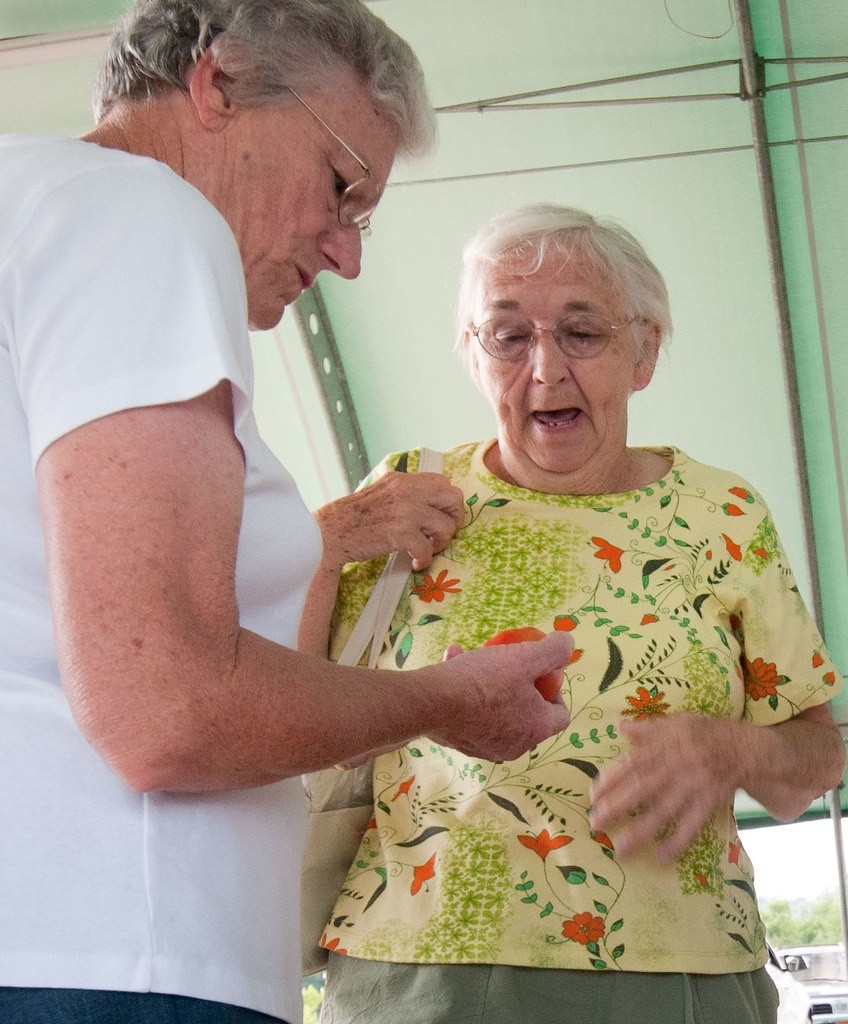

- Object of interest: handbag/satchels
[300,448,444,977]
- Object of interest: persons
[299,201,846,1023]
[1,0,572,1024]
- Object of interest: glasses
[239,34,381,239]
[470,312,639,360]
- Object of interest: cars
[764,938,848,1024]
